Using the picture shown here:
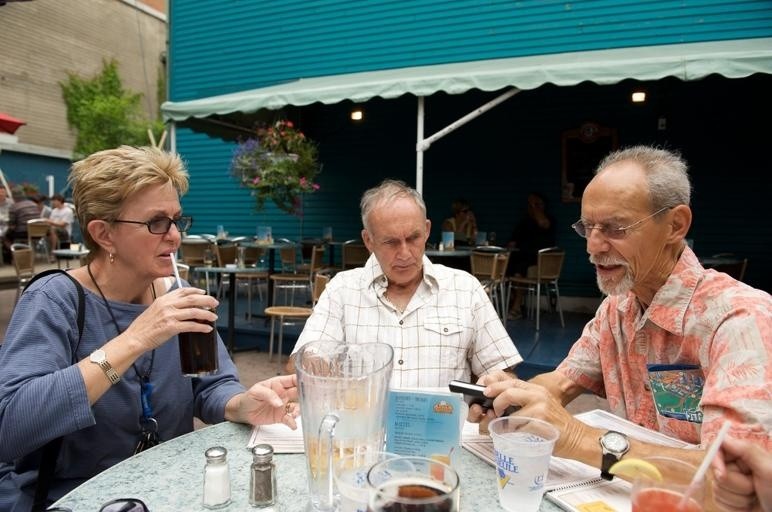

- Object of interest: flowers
[231,115,326,216]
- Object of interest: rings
[286,402,291,413]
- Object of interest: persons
[0,185,74,260]
[1,144,309,512]
[703,436,770,512]
[466,145,771,491]
[284,178,520,389]
[442,201,479,246]
[503,189,553,321]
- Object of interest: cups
[176,307,221,376]
[295,339,395,509]
[491,231,496,246]
[235,246,247,267]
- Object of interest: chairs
[506,247,565,330]
[1,204,91,306]
[426,231,511,325]
[699,252,752,282]
[172,225,369,374]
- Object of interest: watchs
[597,427,631,483]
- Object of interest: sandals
[507,307,524,322]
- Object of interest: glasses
[458,207,472,215]
[571,205,674,241]
[111,214,194,235]
[46,497,151,512]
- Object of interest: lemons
[607,458,664,482]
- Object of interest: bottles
[366,455,460,512]
[486,417,561,510]
[202,445,233,509]
[247,444,278,506]
[627,459,709,512]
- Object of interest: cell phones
[448,379,514,418]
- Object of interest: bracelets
[89,349,121,387]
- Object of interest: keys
[133,417,163,456]
